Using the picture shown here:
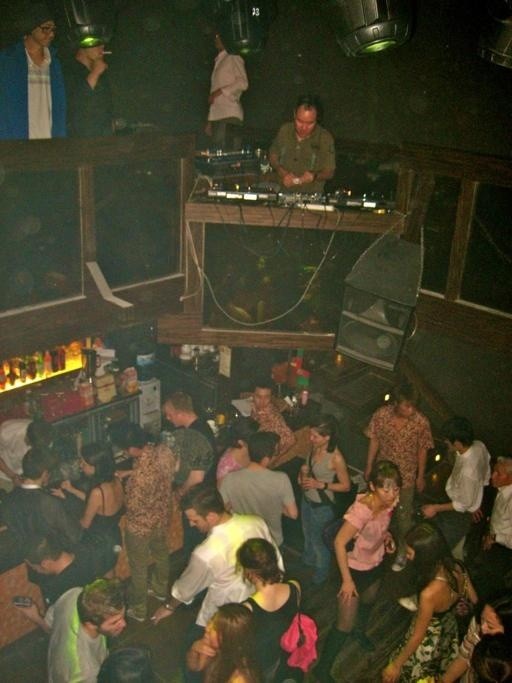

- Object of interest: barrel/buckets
[127,341,156,381]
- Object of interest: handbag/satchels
[332,473,358,516]
[321,518,356,551]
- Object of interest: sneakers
[148,590,166,600]
[391,555,407,571]
[126,609,145,622]
[396,595,418,612]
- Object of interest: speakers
[333,233,425,373]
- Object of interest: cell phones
[13,593,32,609]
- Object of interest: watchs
[164,600,175,611]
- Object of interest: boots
[351,601,377,651]
[312,621,349,683]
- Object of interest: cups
[300,467,309,486]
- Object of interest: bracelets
[323,482,328,490]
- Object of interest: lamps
[59,0,117,48]
[201,0,270,58]
[327,0,416,59]
[475,1,511,71]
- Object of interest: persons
[204,26,251,152]
[150,482,284,679]
[13,538,90,637]
[269,98,338,196]
[44,579,127,679]
[481,456,512,553]
[0,16,67,139]
[364,380,433,547]
[285,414,350,587]
[98,645,164,679]
[61,442,125,594]
[312,461,402,679]
[186,538,301,680]
[205,603,264,679]
[2,448,84,609]
[1,418,54,495]
[215,431,298,559]
[164,391,216,570]
[112,423,174,622]
[216,418,259,486]
[383,520,511,680]
[248,376,296,466]
[65,42,117,137]
[421,417,491,546]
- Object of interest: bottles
[0,346,68,390]
